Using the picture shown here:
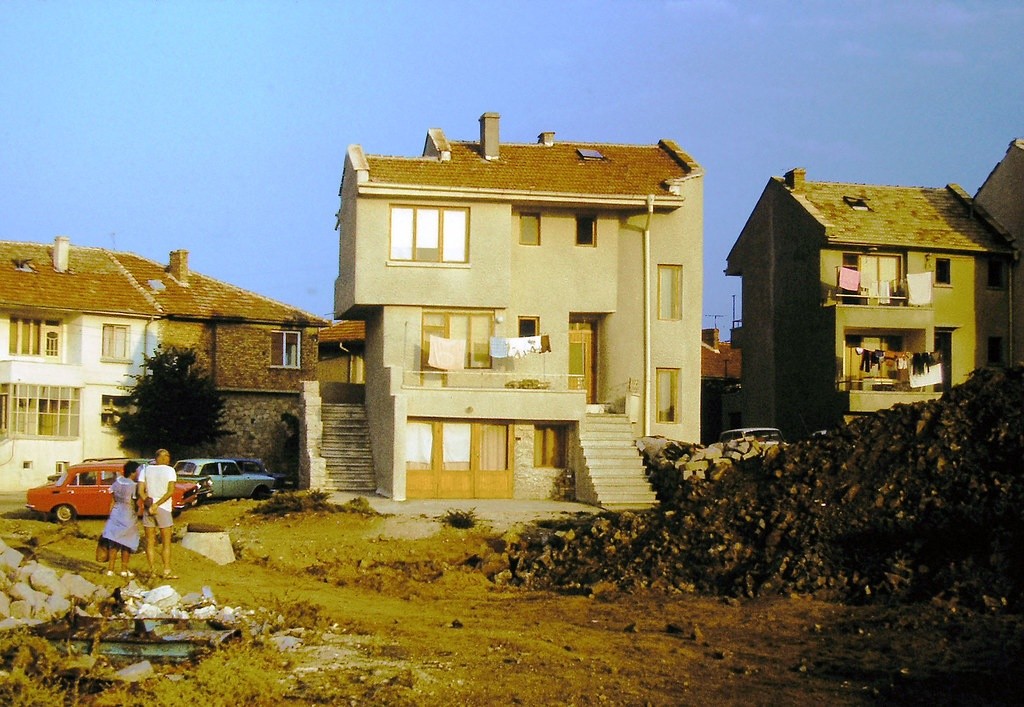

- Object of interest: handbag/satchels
[95,534,110,563]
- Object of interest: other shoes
[147,568,179,579]
[119,569,136,578]
[106,570,116,576]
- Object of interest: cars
[229,457,287,491]
[45,457,214,507]
[172,457,275,504]
[718,427,791,450]
[25,458,199,523]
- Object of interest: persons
[137,448,179,579]
[102,460,142,578]
